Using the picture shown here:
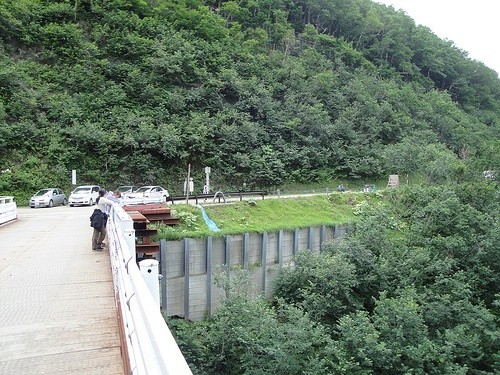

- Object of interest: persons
[90,189,121,251]
[338,183,345,192]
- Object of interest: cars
[68,185,101,207]
[124,185,169,202]
[28,188,67,208]
[110,185,139,198]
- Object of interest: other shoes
[101,243,105,246]
[93,247,103,250]
